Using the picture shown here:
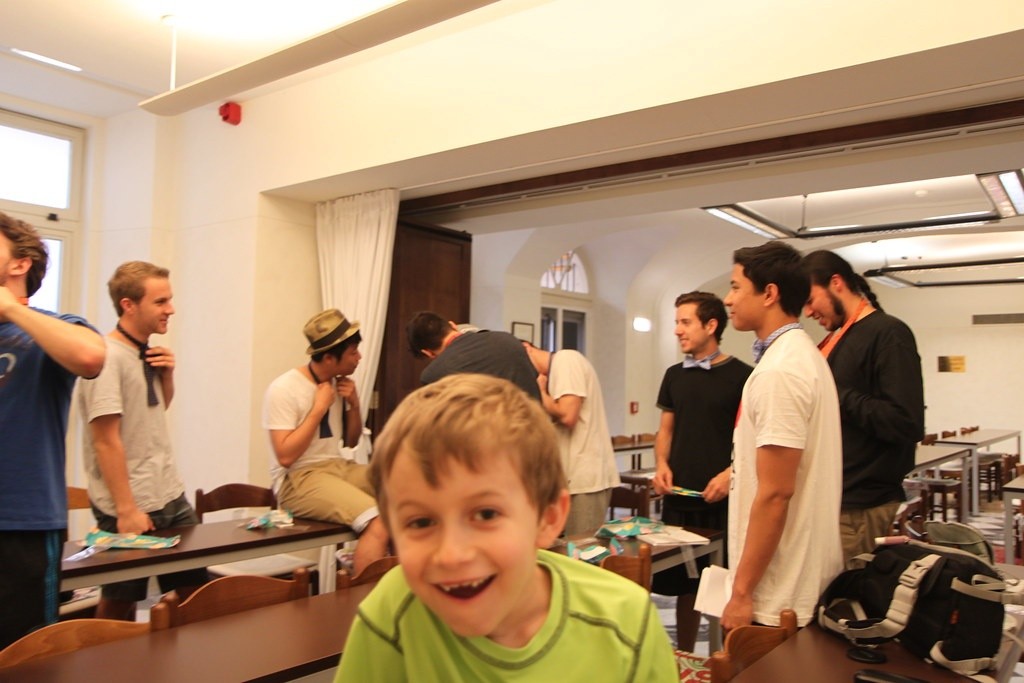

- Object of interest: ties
[116,321,161,407]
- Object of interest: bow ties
[751,321,802,364]
[682,350,721,370]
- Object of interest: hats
[304,308,360,355]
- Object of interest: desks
[0,425,1024,683]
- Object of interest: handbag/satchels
[813,537,1024,683]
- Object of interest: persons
[0,212,106,652]
[719,242,843,633]
[652,290,756,657]
[406,311,621,543]
[806,250,926,575]
[329,373,678,683]
[265,313,390,582]
[79,260,210,621]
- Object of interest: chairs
[0,431,1024,683]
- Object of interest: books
[636,527,710,547]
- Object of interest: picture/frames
[512,322,534,344]
